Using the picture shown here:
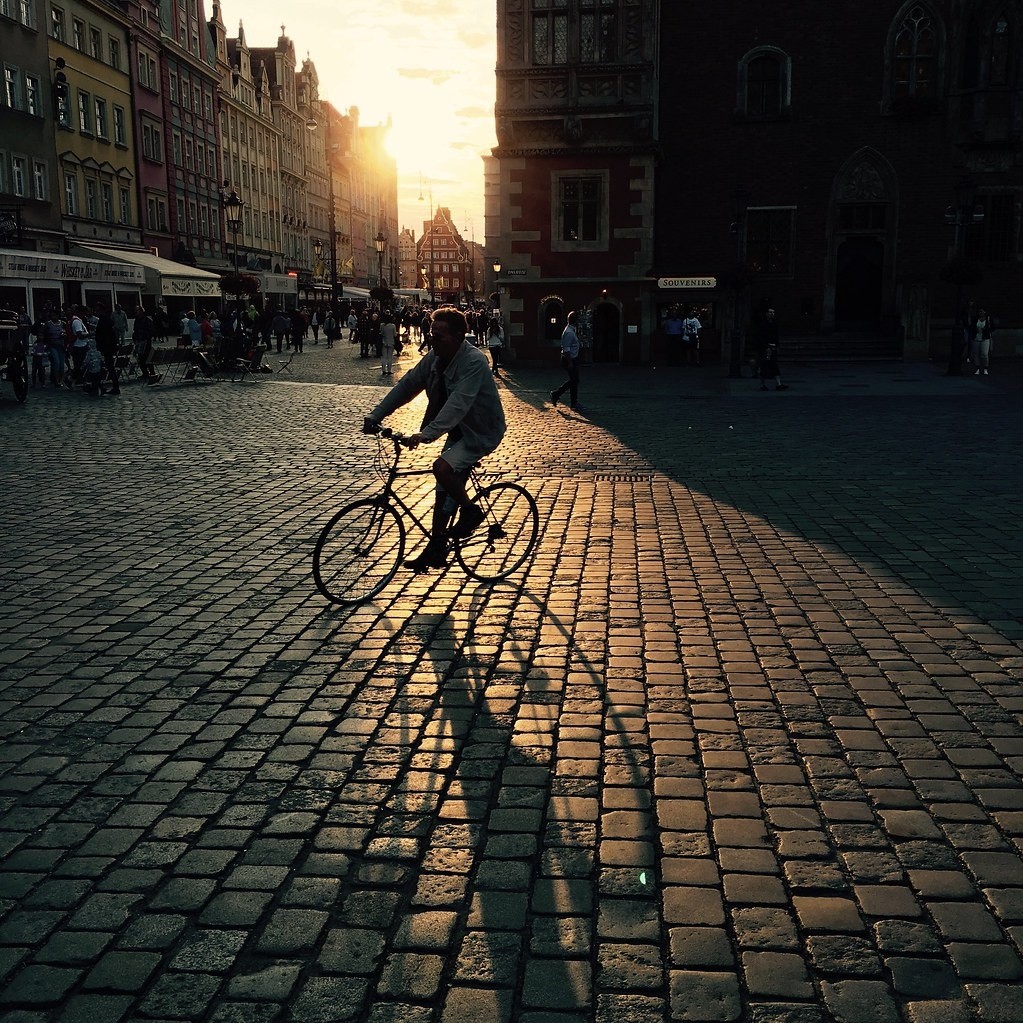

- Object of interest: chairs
[77,341,296,387]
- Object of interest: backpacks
[65,318,78,343]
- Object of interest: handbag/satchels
[990,338,994,352]
[688,333,697,340]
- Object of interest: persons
[0,299,128,396]
[664,312,682,366]
[961,298,976,365]
[681,310,703,367]
[276,298,367,355]
[356,306,403,357]
[365,309,506,569]
[132,301,273,387]
[755,305,789,392]
[972,306,992,376]
[401,299,490,355]
[550,311,586,411]
[486,316,506,379]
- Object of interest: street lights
[374,231,388,316]
[418,178,435,311]
[312,237,323,286]
[223,192,245,324]
[464,210,475,297]
[492,260,502,309]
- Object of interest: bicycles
[312,423,541,607]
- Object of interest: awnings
[68,241,223,317]
[198,262,299,311]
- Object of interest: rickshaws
[0,308,30,402]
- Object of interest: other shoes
[300,351,303,354]
[776,383,789,392]
[571,402,588,410]
[106,388,119,395]
[984,369,988,375]
[41,384,46,389]
[294,349,298,351]
[550,390,558,407]
[386,372,392,375]
[138,374,148,380]
[403,540,448,569]
[383,371,387,374]
[418,349,422,353]
[974,368,980,375]
[448,503,485,538]
[285,347,291,350]
[274,351,281,354]
